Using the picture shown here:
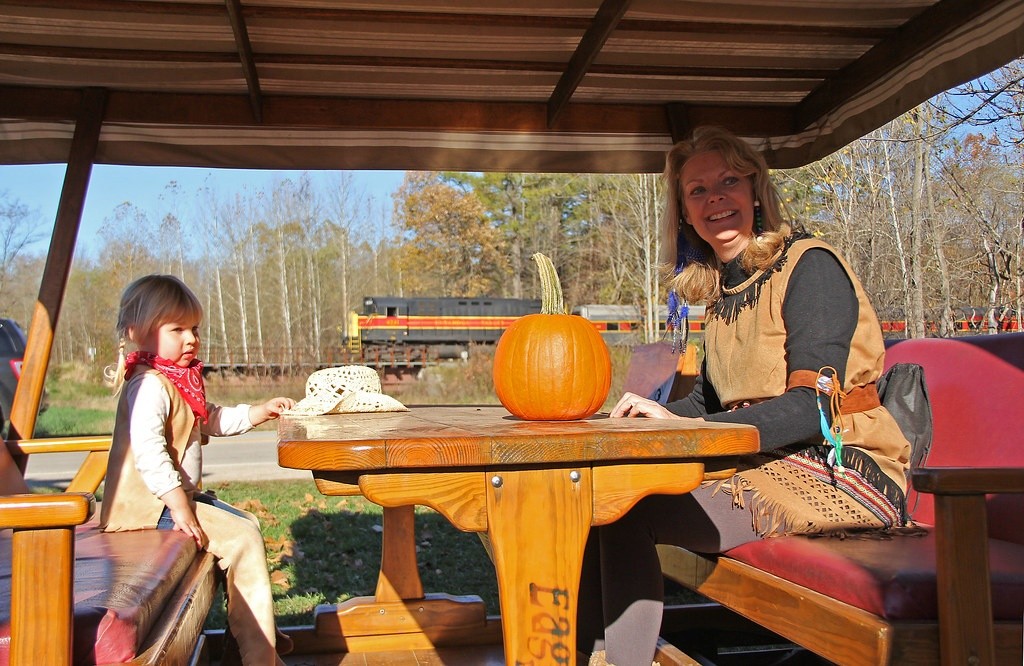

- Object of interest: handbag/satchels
[875,363,933,521]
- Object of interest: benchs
[0,434,219,666]
[653,332,1024,665]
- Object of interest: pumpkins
[493,252,611,421]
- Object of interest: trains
[342,294,1024,384]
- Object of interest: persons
[575,127,928,666]
[99,276,297,666]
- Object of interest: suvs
[1,317,49,436]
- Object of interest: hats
[279,364,412,416]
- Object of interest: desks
[277,404,760,665]
